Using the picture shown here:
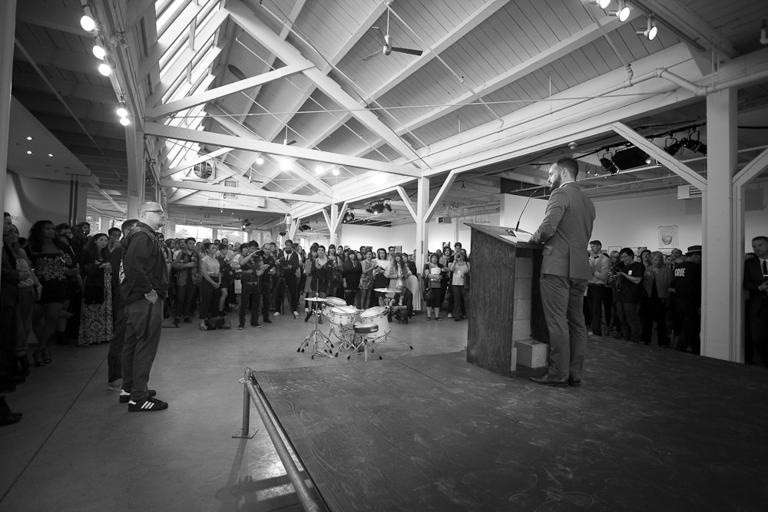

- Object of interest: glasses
[145,208,163,215]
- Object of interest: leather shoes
[529,371,570,388]
[568,370,582,386]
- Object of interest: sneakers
[426,317,431,321]
[118,384,157,403]
[263,320,272,325]
[447,312,467,321]
[293,311,299,316]
[273,311,280,316]
[435,317,442,321]
[239,324,244,329]
[305,307,322,317]
[251,322,262,328]
[174,316,192,328]
[128,396,169,412]
[107,378,124,392]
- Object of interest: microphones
[515,188,540,231]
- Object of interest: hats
[455,242,461,247]
[685,245,701,255]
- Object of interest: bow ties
[594,256,600,259]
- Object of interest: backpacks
[110,222,156,302]
[245,275,258,296]
[84,260,110,305]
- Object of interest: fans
[361,7,423,61]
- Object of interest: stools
[347,324,382,362]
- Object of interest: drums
[329,306,357,325]
[361,307,391,339]
[332,309,365,341]
[322,297,347,318]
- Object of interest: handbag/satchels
[233,273,243,295]
[259,264,274,296]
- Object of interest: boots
[0,353,31,426]
[31,350,48,366]
[40,348,52,364]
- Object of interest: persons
[582,239,701,356]
[108,219,140,392]
[117,201,167,410]
[2,217,122,391]
[742,235,767,365]
[423,241,470,320]
[154,232,420,331]
[528,157,594,386]
[0,211,30,426]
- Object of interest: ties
[763,259,768,280]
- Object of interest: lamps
[609,0,630,22]
[242,219,251,229]
[684,127,707,154]
[279,198,392,236]
[599,147,618,174]
[591,0,611,8]
[636,14,657,41]
[658,134,685,164]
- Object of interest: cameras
[612,266,620,275]
[456,255,460,258]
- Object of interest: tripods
[297,303,360,360]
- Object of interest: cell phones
[257,251,264,255]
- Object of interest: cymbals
[304,298,328,302]
[374,287,403,292]
